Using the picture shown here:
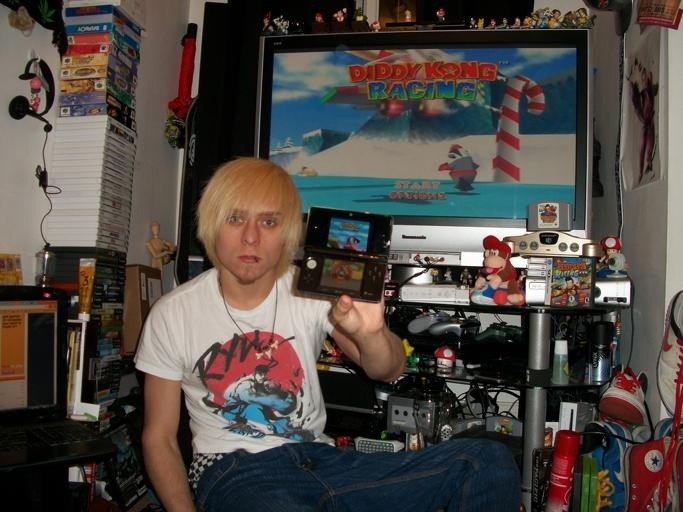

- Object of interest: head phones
[9,58,55,120]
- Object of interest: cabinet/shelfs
[172,244,609,512]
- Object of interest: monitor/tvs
[253,29,593,269]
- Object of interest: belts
[187,452,225,489]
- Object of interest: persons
[133,157,522,511]
[144,218,174,272]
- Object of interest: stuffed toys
[597,235,622,264]
[469,235,526,306]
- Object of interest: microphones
[27,109,52,131]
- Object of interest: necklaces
[217,276,280,354]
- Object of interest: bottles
[551,340,569,385]
[589,321,614,382]
[545,430,580,512]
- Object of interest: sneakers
[655,289,682,422]
[599,363,647,426]
[582,418,682,511]
